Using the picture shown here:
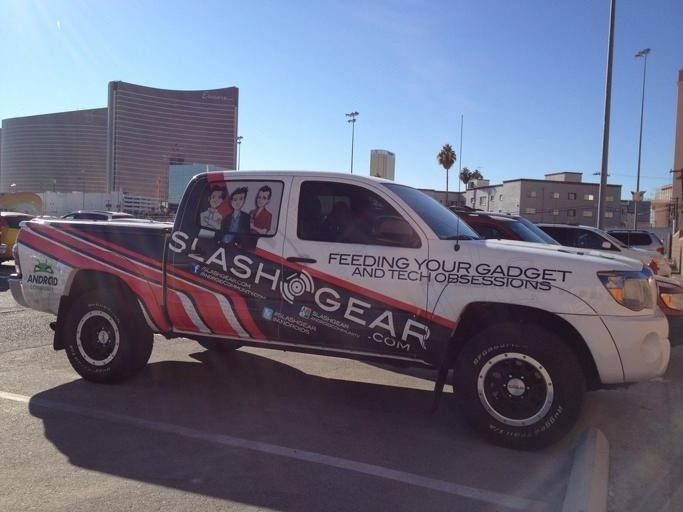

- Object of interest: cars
[448,207,681,348]
[0,209,36,262]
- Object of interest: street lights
[233,135,246,173]
[343,110,363,175]
[630,47,651,229]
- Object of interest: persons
[221,186,250,233]
[200,184,228,230]
[248,185,272,234]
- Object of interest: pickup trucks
[6,168,672,450]
